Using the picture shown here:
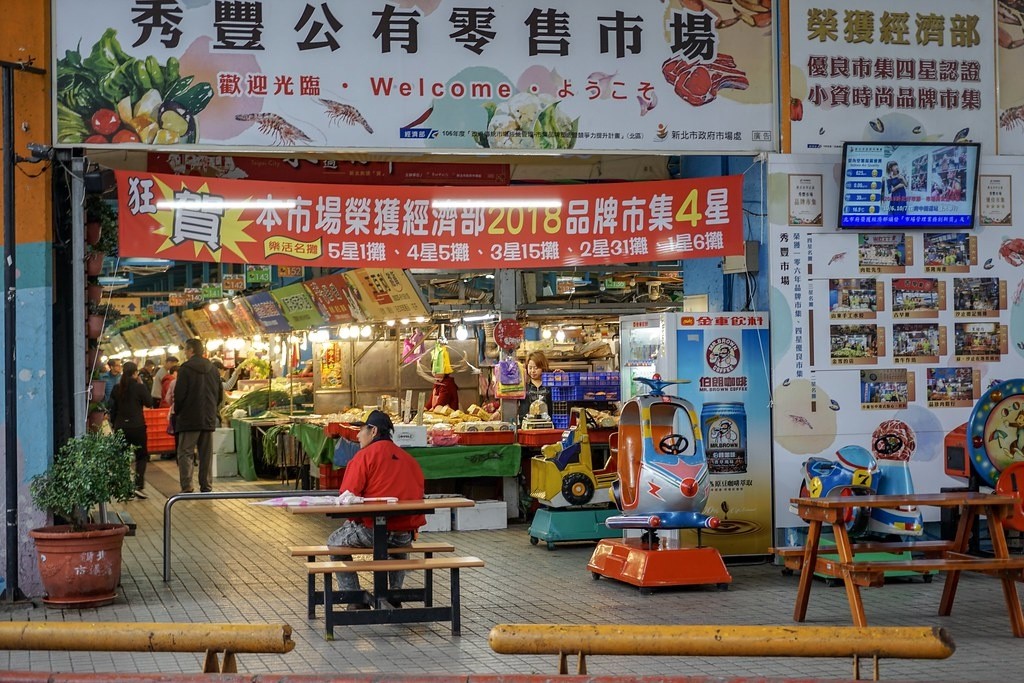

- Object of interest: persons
[926,242,965,267]
[139,356,198,466]
[211,358,243,428]
[886,160,907,214]
[877,385,907,402]
[964,332,992,348]
[174,338,223,493]
[953,282,998,310]
[423,362,459,412]
[518,352,566,501]
[107,362,152,498]
[912,338,939,356]
[929,380,973,399]
[911,151,967,200]
[849,291,870,307]
[881,177,885,205]
[903,295,921,311]
[327,410,427,611]
[831,337,878,356]
[859,244,903,266]
[99,359,122,429]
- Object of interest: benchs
[289,543,485,637]
[767,539,1024,637]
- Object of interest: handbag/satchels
[166,413,174,435]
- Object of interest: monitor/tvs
[837,141,981,230]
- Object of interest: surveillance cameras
[14,142,55,163]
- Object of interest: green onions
[219,387,287,422]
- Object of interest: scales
[521,417,555,430]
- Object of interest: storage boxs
[319,462,345,491]
[326,421,362,442]
[212,429,238,478]
[420,493,508,532]
[237,379,268,393]
[386,370,621,446]
[143,408,177,453]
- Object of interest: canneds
[700,401,748,474]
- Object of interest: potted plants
[84,197,121,434]
[30,432,140,610]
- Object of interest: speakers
[84,162,117,195]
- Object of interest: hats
[349,410,395,434]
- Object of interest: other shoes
[346,603,371,610]
[388,597,402,610]
[134,489,147,499]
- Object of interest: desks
[526,445,609,470]
[791,492,1023,637]
[234,410,313,481]
[285,496,475,608]
[333,437,522,524]
[288,422,339,491]
